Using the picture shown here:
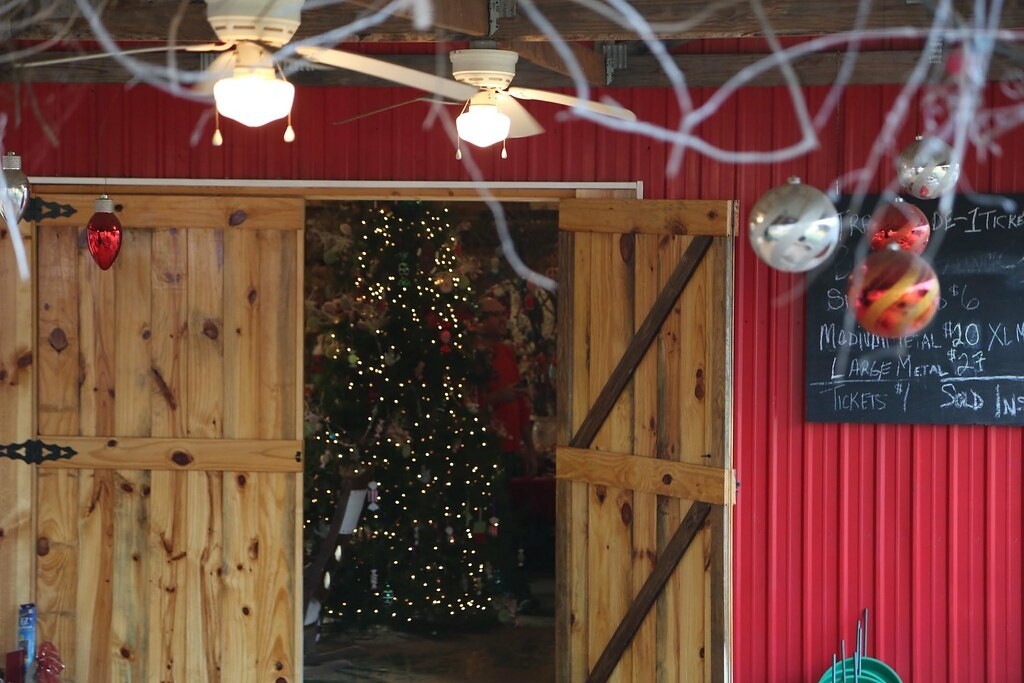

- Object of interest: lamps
[212,66,294,148]
[456,103,512,162]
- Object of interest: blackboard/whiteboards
[800,186,1024,429]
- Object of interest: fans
[15,1,481,102]
[333,46,637,139]
